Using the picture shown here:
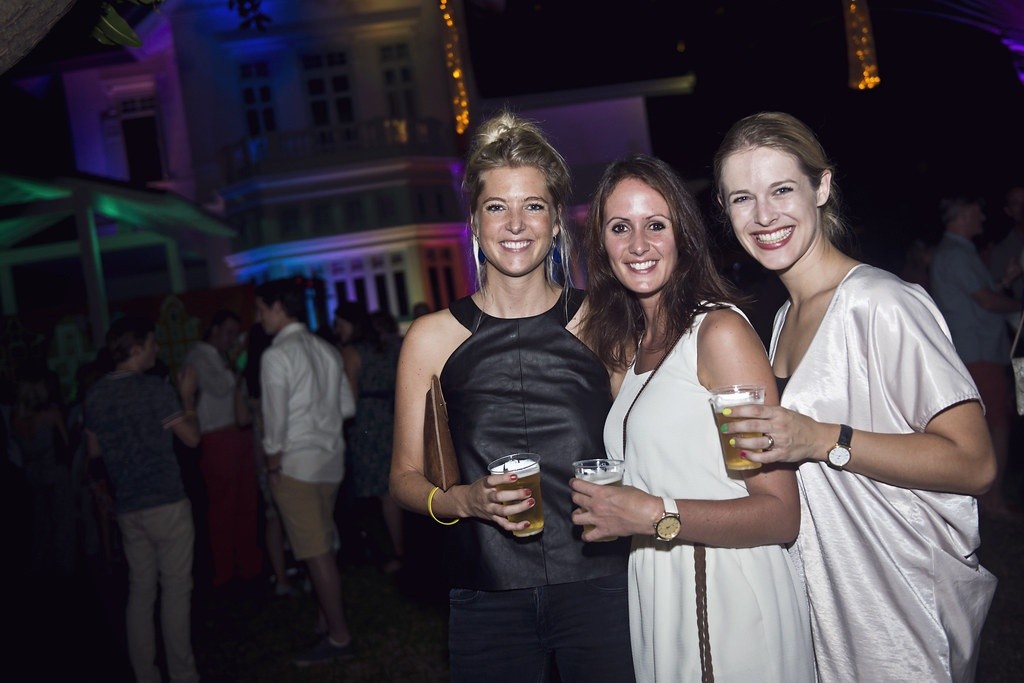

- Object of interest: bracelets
[427,486,460,525]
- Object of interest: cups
[571,458,624,542]
[707,383,766,471]
[488,452,545,537]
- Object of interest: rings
[763,436,775,451]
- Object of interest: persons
[251,279,358,665]
[568,153,820,683]
[86,315,211,683]
[719,113,1000,683]
[4,261,431,614]
[382,128,639,683]
[926,181,1018,522]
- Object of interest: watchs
[825,424,854,472]
[650,495,683,542]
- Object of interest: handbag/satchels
[1010,312,1024,417]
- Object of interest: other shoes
[292,630,355,666]
